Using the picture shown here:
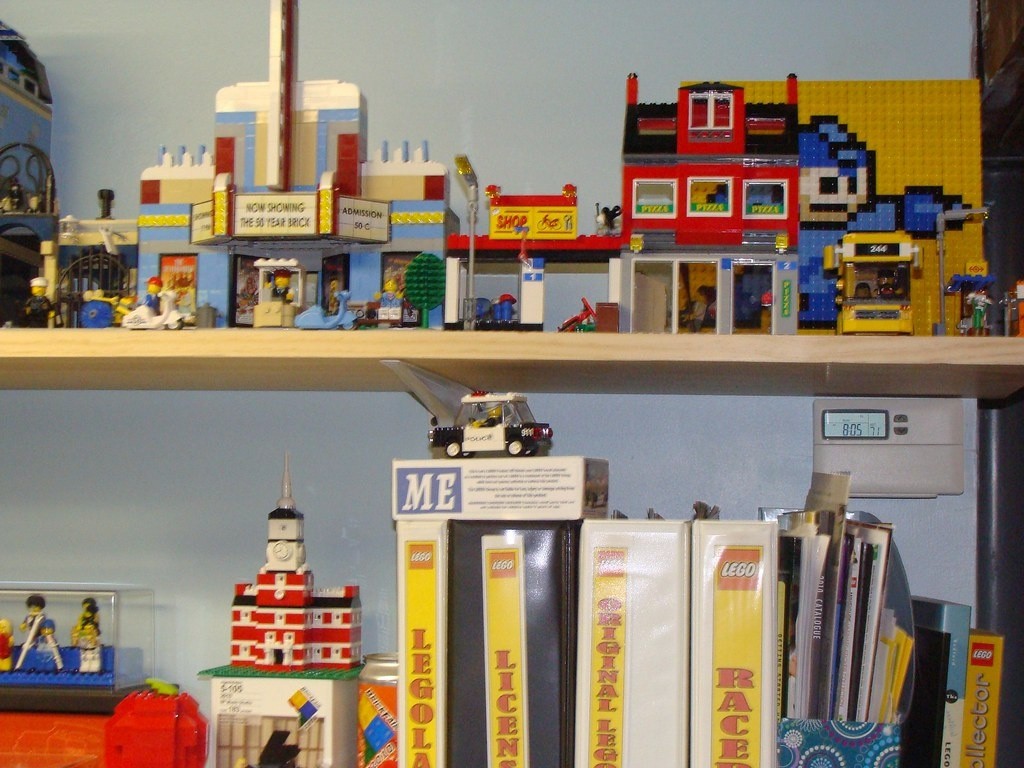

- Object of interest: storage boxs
[0,582,155,713]
[392,457,610,519]
[757,507,916,768]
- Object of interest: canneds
[354,652,400,768]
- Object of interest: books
[393,473,1005,768]
